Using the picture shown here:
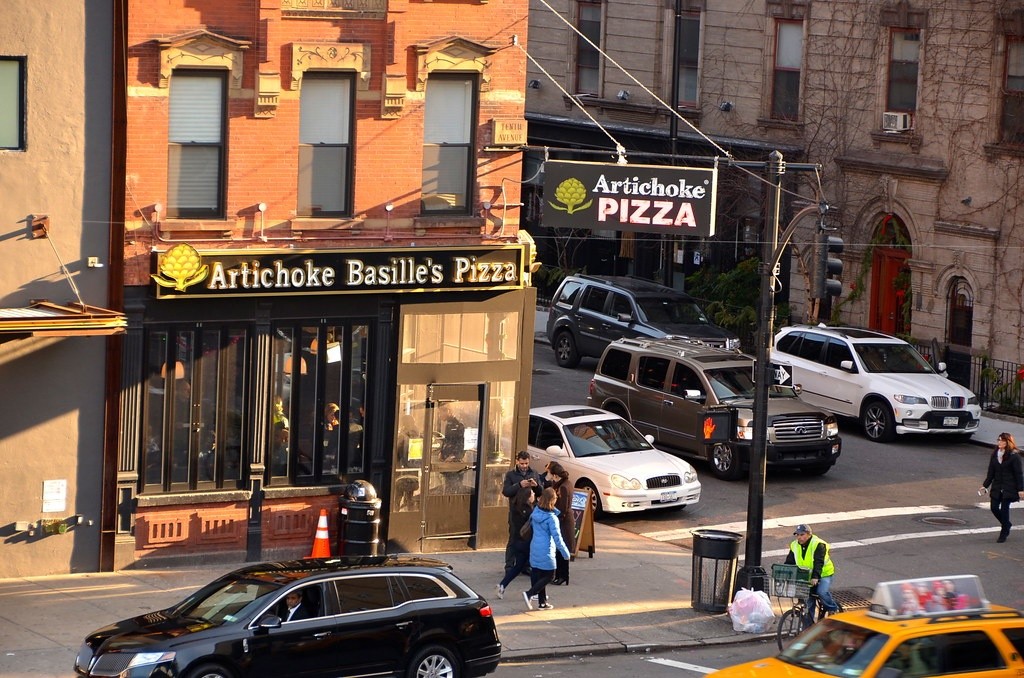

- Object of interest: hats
[793,524,812,534]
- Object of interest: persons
[978,433,1024,543]
[175,380,190,401]
[274,422,288,466]
[501,451,543,579]
[325,401,365,431]
[539,460,559,486]
[496,557,549,600]
[782,524,838,628]
[522,487,570,610]
[550,464,576,585]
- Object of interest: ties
[286,610,290,619]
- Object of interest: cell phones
[528,479,532,482]
[978,489,989,496]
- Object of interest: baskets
[773,578,810,599]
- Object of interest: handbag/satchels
[520,510,534,540]
[1003,490,1019,502]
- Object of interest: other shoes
[495,583,503,600]
[522,590,534,612]
[996,522,1013,543]
[523,567,532,575]
[539,603,554,610]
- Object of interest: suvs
[587,334,842,479]
[702,575,1024,678]
[546,272,742,368]
[769,321,980,442]
[75,556,501,677]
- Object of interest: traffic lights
[810,232,845,299]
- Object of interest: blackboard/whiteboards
[569,488,595,556]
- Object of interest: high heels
[551,575,569,586]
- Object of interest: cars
[499,403,702,520]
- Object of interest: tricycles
[769,578,877,652]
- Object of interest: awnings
[0,300,129,338]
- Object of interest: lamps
[915,291,923,312]
[720,102,732,113]
[528,80,541,89]
[617,90,629,99]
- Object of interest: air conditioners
[883,112,911,131]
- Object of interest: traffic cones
[303,509,332,560]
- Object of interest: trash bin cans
[689,529,743,613]
[338,479,381,556]
